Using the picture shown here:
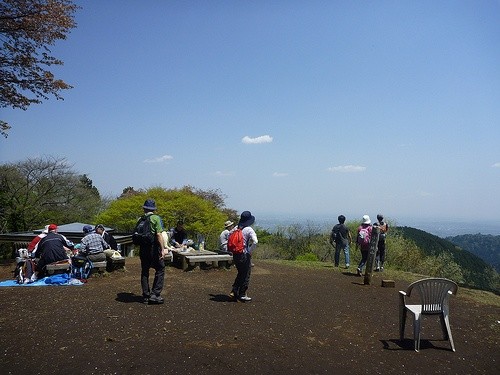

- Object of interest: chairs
[398,277,459,353]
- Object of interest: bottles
[199,243,202,252]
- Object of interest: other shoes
[143,296,149,303]
[376,267,379,271]
[345,264,349,268]
[229,292,241,303]
[380,267,383,271]
[149,293,165,304]
[240,293,252,300]
[251,263,255,266]
[357,268,362,277]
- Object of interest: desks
[172,249,218,271]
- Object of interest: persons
[355,215,373,277]
[330,216,353,269]
[219,220,235,252]
[135,198,168,304]
[373,215,388,271]
[27,223,119,278]
[230,211,259,301]
[170,220,188,247]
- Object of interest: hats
[377,214,384,220]
[224,220,234,227]
[92,224,103,231]
[82,226,95,235]
[48,224,60,229]
[43,225,50,233]
[143,199,158,210]
[239,211,255,226]
[361,215,371,224]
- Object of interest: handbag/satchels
[19,248,28,258]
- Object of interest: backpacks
[227,224,250,254]
[132,214,158,245]
[358,226,371,245]
[377,222,387,238]
[16,259,40,285]
[331,224,346,244]
[67,256,93,282]
[12,261,25,277]
[45,273,70,286]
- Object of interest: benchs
[185,255,233,269]
[46,261,107,276]
